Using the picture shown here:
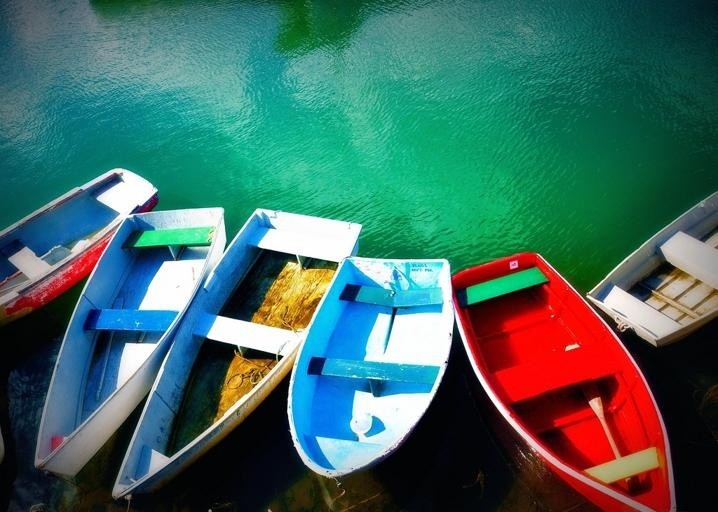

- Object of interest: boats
[110,207,365,501]
[451,250,675,511]
[0,166,159,323]
[33,205,228,479]
[286,255,456,478]
[584,192,718,351]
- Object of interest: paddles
[582,381,631,482]
